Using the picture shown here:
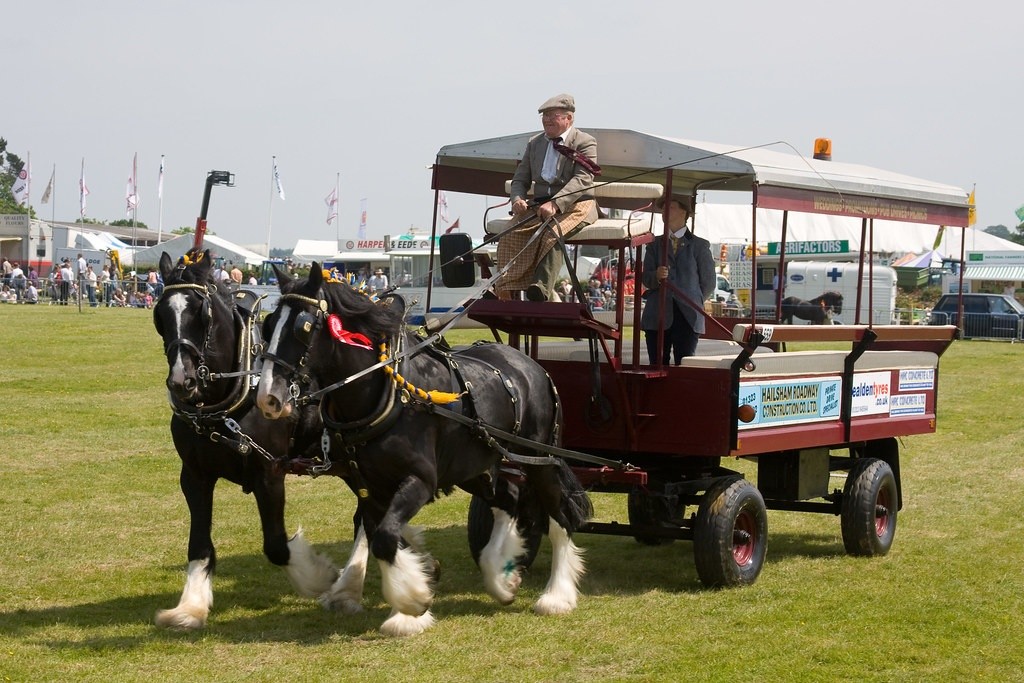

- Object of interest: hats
[538,93,576,112]
[374,268,384,274]
[657,194,693,212]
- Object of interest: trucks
[702,273,742,318]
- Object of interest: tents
[890,249,946,268]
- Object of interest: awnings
[962,265,1024,282]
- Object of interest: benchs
[422,311,961,378]
[482,179,664,374]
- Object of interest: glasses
[536,112,564,120]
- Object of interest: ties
[549,137,603,177]
[670,237,678,256]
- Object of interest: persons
[481,93,599,302]
[557,278,617,311]
[641,192,716,365]
[0,253,412,310]
[705,294,744,318]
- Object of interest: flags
[10,158,30,208]
[436,189,450,224]
[126,154,142,217]
[80,165,89,218]
[325,176,339,226]
[446,217,460,235]
[272,159,286,201]
[41,170,54,204]
[156,156,166,198]
[357,200,367,239]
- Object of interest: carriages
[153,126,978,641]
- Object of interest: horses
[781,291,843,326]
[150,248,587,640]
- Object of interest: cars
[930,292,1024,342]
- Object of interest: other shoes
[482,288,497,301]
[525,284,546,302]
